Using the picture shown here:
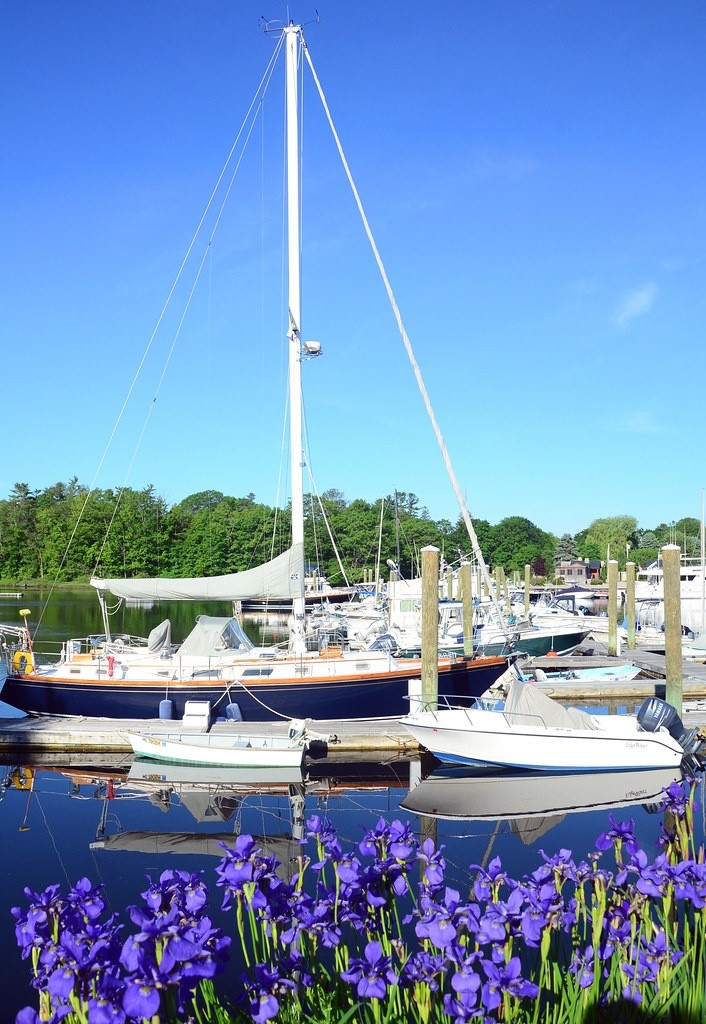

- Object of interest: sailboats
[0,23,706,750]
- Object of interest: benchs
[232,740,249,748]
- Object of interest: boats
[113,713,340,769]
[126,757,332,844]
[398,753,706,839]
[398,680,701,772]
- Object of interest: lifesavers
[14,651,34,674]
[12,767,33,789]
